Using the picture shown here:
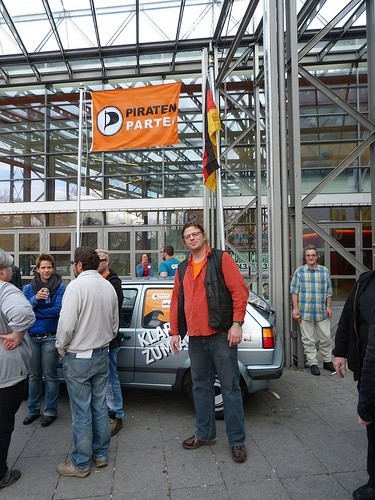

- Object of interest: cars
[42,280,288,420]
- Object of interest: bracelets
[233,323,242,326]
[327,304,330,306]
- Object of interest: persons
[73,249,124,436]
[23,253,67,427]
[136,254,153,277]
[168,220,250,464]
[263,283,269,300]
[9,265,23,292]
[332,270,375,500]
[0,248,36,489]
[158,246,182,276]
[290,245,336,377]
[55,247,119,477]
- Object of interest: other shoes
[108,411,124,437]
[323,361,336,372]
[353,484,375,500]
[0,470,21,488]
[310,365,321,376]
[23,414,41,424]
[40,416,56,427]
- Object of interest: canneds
[43,289,50,303]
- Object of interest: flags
[91,82,181,152]
[202,79,223,194]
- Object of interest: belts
[29,331,56,338]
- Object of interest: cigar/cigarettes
[331,372,336,375]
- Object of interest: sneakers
[183,435,217,450]
[92,457,108,468]
[56,460,90,478]
[232,445,247,463]
